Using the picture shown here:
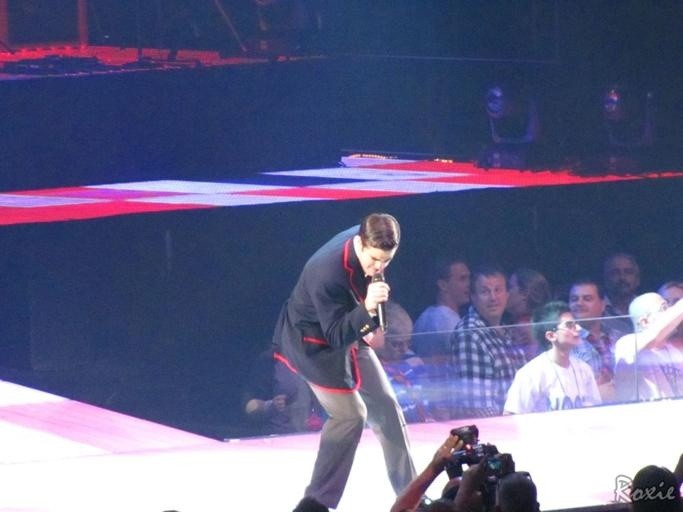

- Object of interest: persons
[629,464,683,512]
[240,248,683,424]
[388,433,540,512]
[271,210,417,512]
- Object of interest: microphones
[371,273,388,333]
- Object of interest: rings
[442,445,446,449]
